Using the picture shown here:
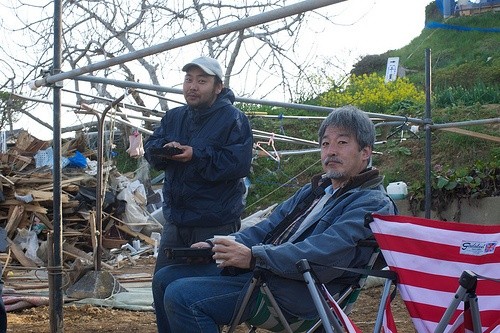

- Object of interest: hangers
[257,132,281,164]
[255,157,299,186]
[386,116,420,141]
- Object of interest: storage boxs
[386,181,408,200]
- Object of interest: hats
[182,56,223,81]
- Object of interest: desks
[163,196,398,333]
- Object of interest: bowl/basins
[164,247,213,265]
[146,147,184,158]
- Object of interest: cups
[214,235,235,263]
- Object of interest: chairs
[296,213,500,333]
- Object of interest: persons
[141,56,254,332]
[152,105,399,333]
